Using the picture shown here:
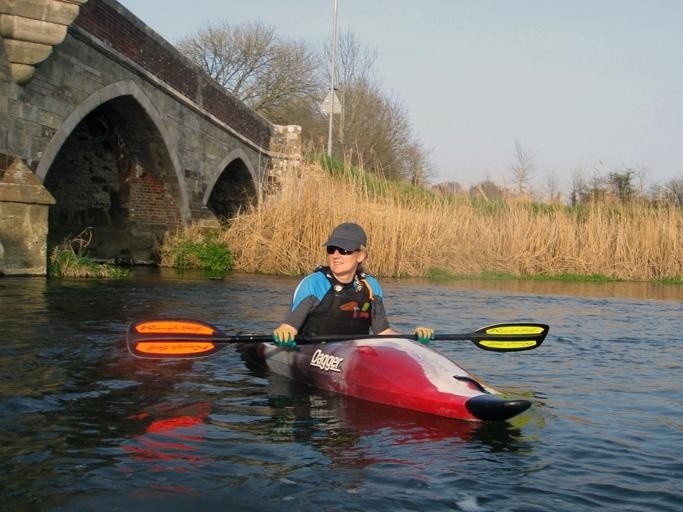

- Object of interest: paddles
[125,318,549,361]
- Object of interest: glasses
[327,246,361,255]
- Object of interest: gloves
[272,323,299,348]
[409,327,435,344]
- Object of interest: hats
[322,223,367,252]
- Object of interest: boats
[236,328,533,424]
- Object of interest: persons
[271,222,436,349]
